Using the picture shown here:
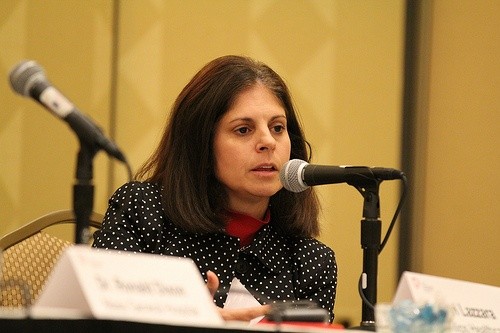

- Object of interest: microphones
[8,57,126,164]
[280,159,404,193]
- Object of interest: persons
[91,55,337,326]
